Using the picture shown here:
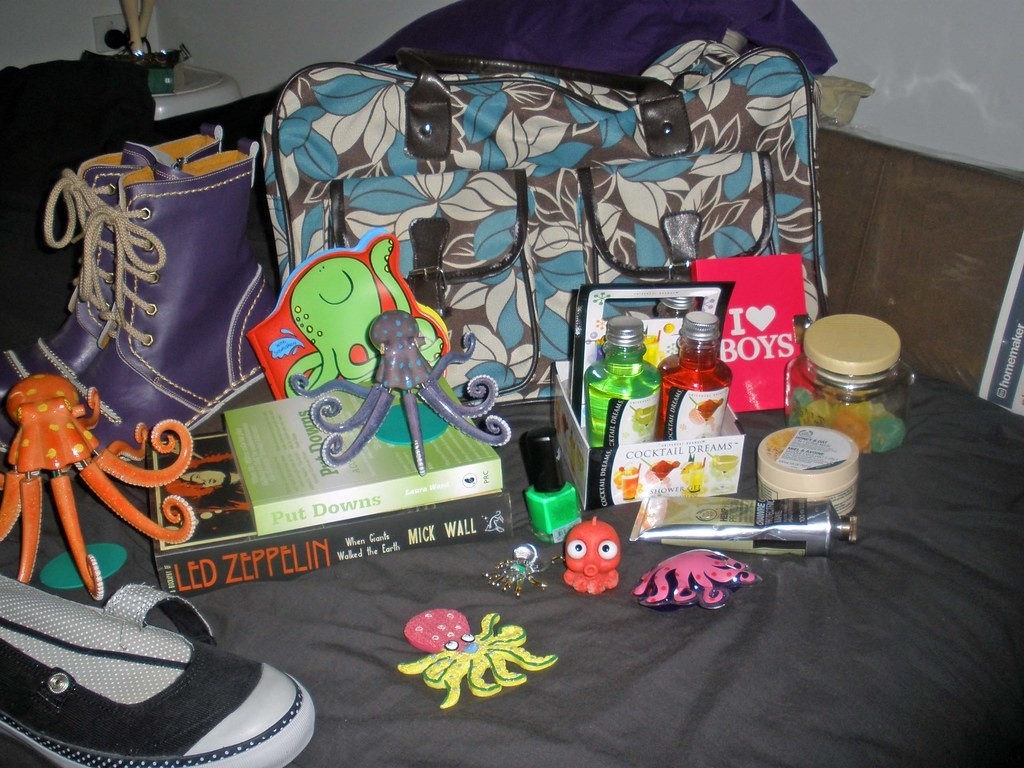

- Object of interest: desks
[152,64,243,121]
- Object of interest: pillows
[0,51,161,352]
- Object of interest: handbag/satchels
[261,26,833,408]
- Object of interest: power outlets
[93,13,126,52]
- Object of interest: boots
[0,125,282,475]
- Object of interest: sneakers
[0,574,318,768]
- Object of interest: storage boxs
[551,360,744,511]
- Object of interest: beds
[0,341,1023,767]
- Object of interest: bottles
[653,310,733,441]
[583,314,662,447]
